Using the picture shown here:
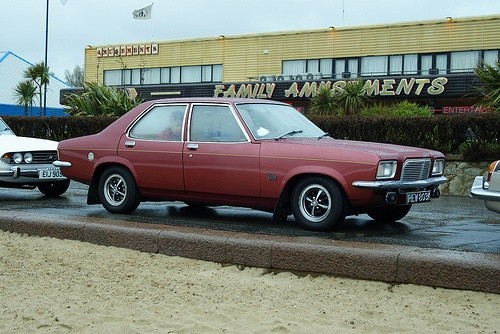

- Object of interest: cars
[53,96,449,234]
[0,114,71,197]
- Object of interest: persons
[153,110,184,142]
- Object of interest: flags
[131,4,152,20]
